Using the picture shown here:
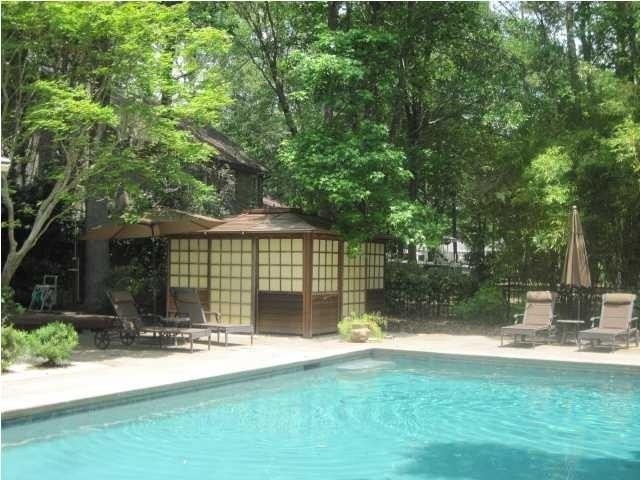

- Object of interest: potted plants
[29,321,80,368]
[337,311,387,343]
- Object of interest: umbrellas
[78,199,227,337]
[560,204,594,339]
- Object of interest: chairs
[103,287,254,352]
[500,290,639,354]
